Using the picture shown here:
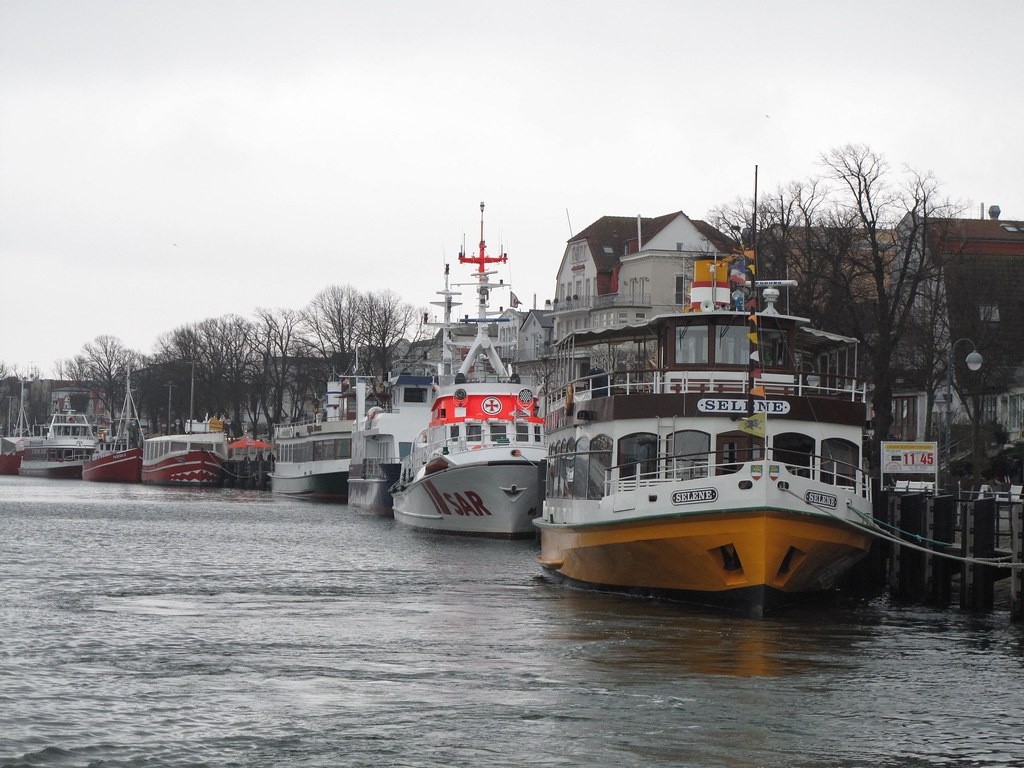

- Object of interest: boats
[267,202,546,541]
[0,360,225,487]
[530,165,878,611]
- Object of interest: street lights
[943,338,983,494]
